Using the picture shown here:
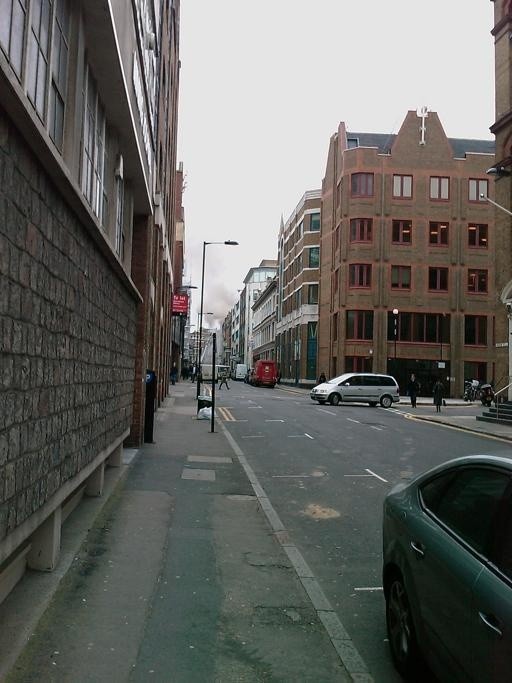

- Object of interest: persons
[219,369,230,389]
[472,378,480,398]
[182,363,199,383]
[319,373,326,384]
[406,373,420,408]
[432,380,445,412]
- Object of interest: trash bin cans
[146,370,157,444]
[198,396,213,420]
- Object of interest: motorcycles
[464,380,495,407]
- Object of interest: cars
[243,368,255,384]
[382,454,511,683]
[310,372,400,408]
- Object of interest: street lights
[196,238,241,400]
[392,308,399,378]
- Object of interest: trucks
[230,364,248,381]
[249,360,278,389]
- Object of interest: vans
[200,365,231,384]
